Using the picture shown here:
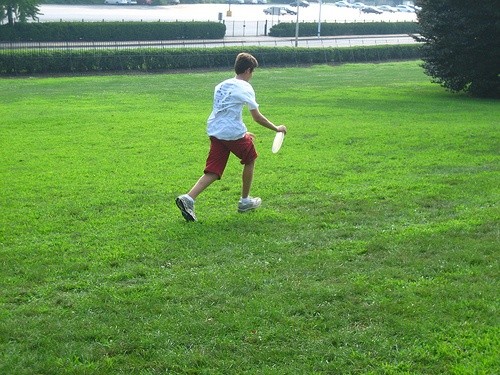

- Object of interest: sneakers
[238,196,262,211]
[175,194,199,223]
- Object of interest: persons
[175,53,287,222]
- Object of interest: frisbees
[272,132,284,154]
